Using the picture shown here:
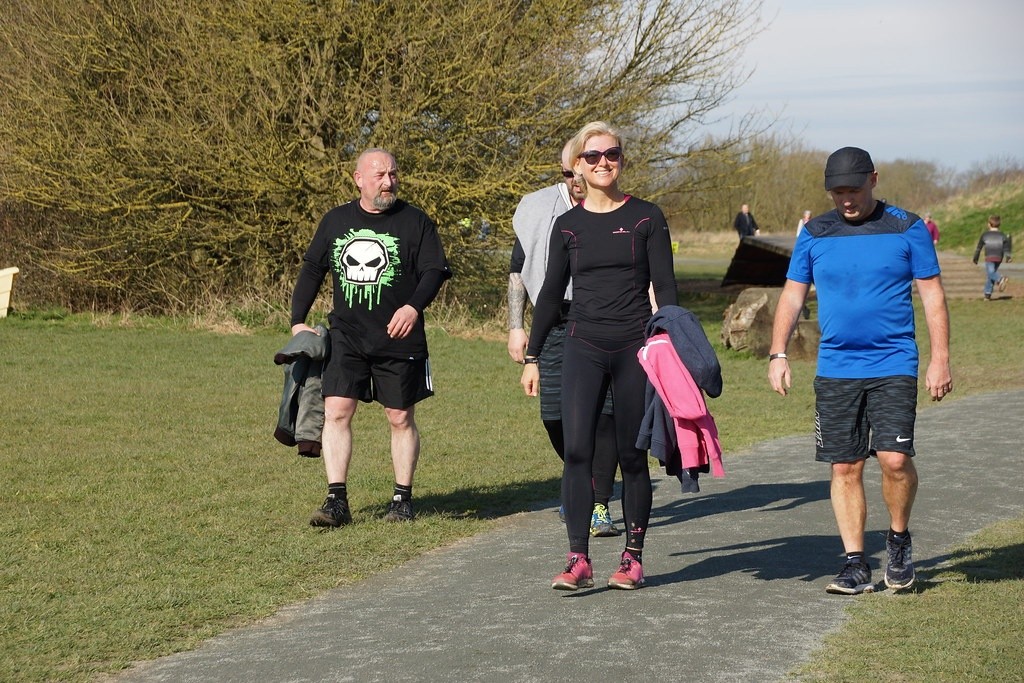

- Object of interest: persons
[973,216,1012,301]
[924,214,939,244]
[735,203,761,239]
[520,122,680,591]
[508,140,619,536]
[768,147,953,594]
[796,210,812,237]
[292,149,450,526]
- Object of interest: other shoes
[999,277,1009,292]
[984,295,989,301]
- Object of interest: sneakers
[589,504,618,537]
[385,501,413,522]
[552,552,594,589]
[559,506,565,522]
[310,494,352,526]
[885,532,915,588]
[608,552,647,590]
[826,558,872,594]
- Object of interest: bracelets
[523,358,539,364]
[769,353,787,361]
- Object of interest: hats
[825,147,875,191]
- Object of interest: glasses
[577,147,622,165]
[562,168,574,178]
